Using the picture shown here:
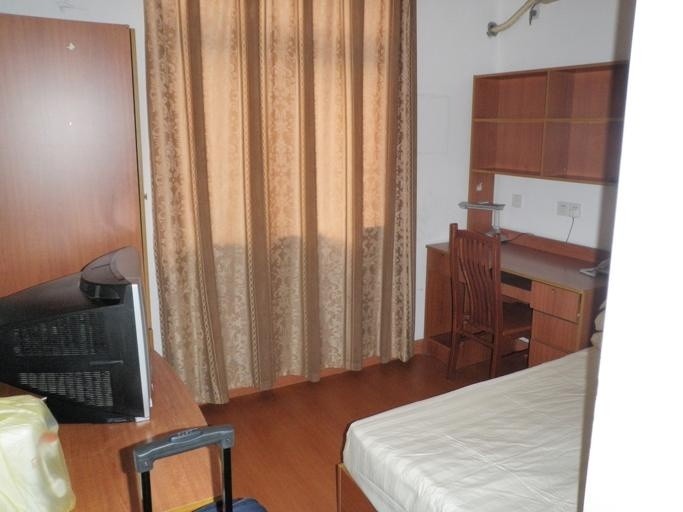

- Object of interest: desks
[422,236,611,374]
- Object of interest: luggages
[133,424,269,511]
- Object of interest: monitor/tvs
[0,246,151,424]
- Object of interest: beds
[333,344,602,512]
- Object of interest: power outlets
[567,202,579,219]
[555,200,567,218]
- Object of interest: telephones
[580,257,609,276]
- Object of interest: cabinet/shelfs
[466,56,631,268]
[0,12,145,371]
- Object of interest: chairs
[445,223,529,381]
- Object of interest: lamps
[457,202,507,246]
